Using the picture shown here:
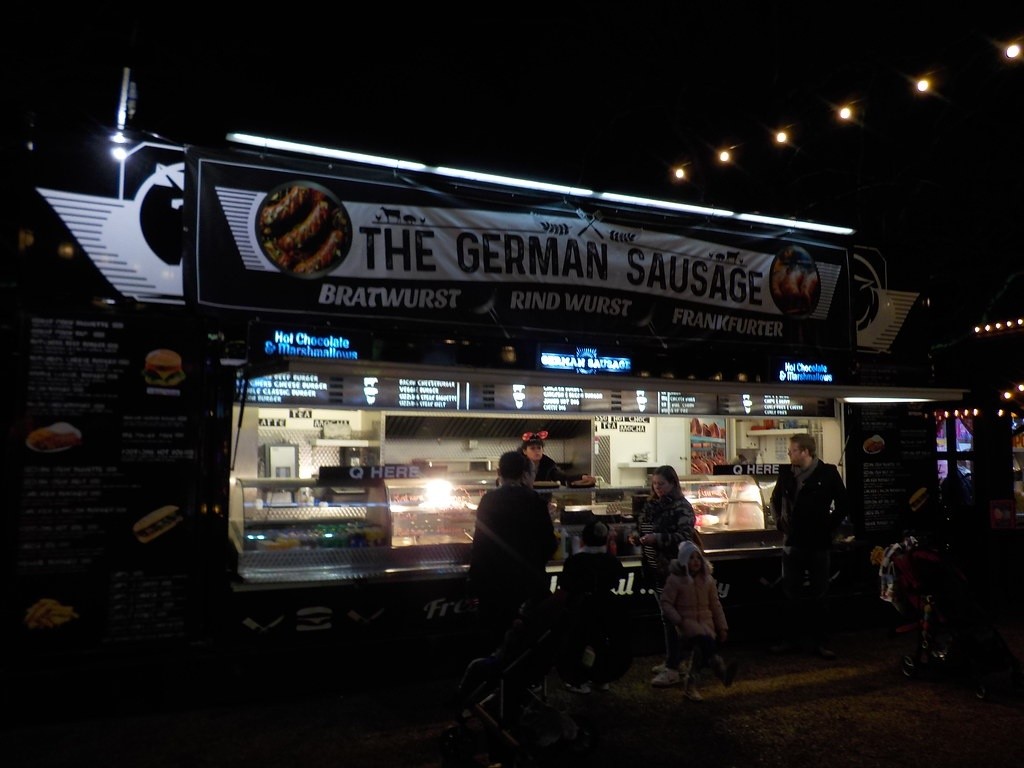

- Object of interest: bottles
[572,535,581,555]
[553,527,571,561]
[779,419,785,429]
[610,535,616,556]
[245,522,386,552]
[623,525,631,544]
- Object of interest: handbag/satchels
[686,526,703,553]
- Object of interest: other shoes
[649,667,689,685]
[591,679,610,691]
[565,679,588,694]
[652,663,666,672]
[770,640,801,653]
[718,655,742,685]
[818,641,838,659]
[681,686,702,700]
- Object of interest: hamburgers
[143,349,187,386]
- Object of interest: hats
[677,540,709,583]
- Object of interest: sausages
[783,270,818,306]
[260,185,345,273]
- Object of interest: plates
[26,440,73,452]
[254,179,353,280]
[768,244,821,317]
[863,437,884,454]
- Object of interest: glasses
[788,448,802,453]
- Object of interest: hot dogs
[909,487,930,511]
[132,506,183,543]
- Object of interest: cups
[764,420,773,430]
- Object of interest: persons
[467,433,728,696]
[770,433,850,658]
